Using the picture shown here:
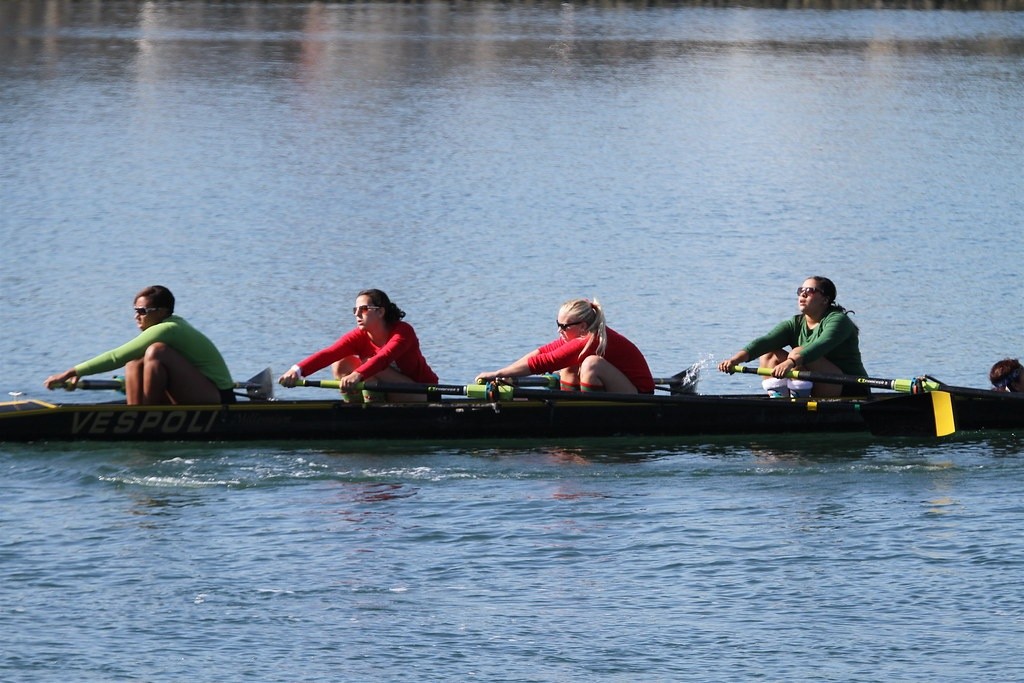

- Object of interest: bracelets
[789,357,796,368]
[291,365,301,378]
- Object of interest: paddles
[281,379,513,400]
[476,364,700,396]
[730,364,940,394]
[50,368,274,402]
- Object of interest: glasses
[134,307,162,315]
[556,319,582,331]
[797,287,824,297]
[353,305,379,315]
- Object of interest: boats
[0,377,1023,448]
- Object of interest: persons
[278,289,441,403]
[718,276,871,398]
[475,298,655,395]
[44,286,236,404]
